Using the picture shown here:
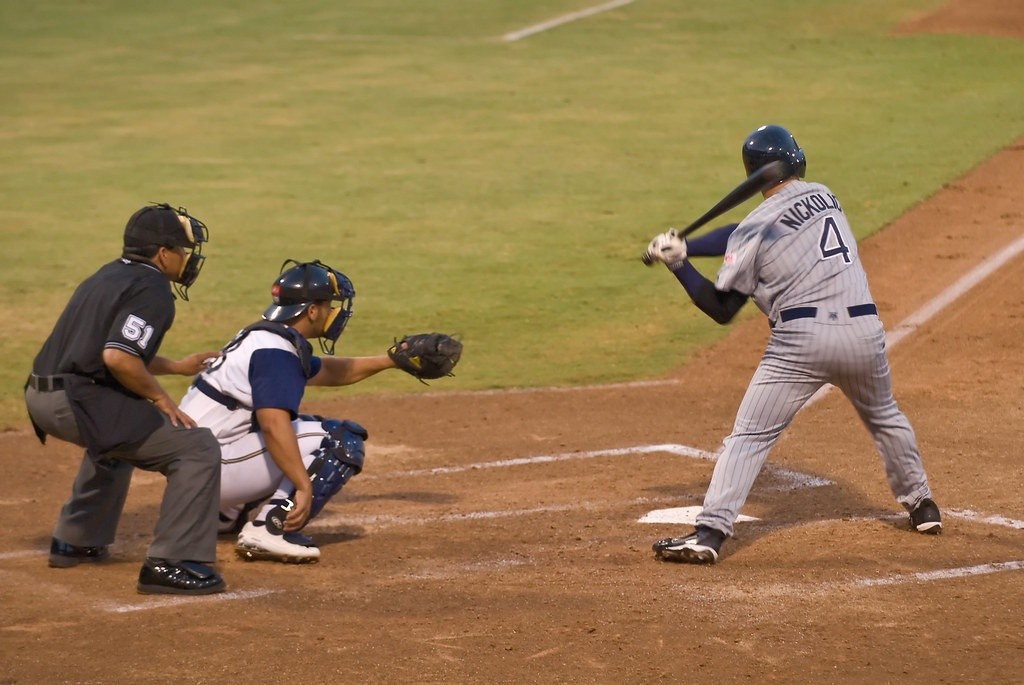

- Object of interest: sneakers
[910,498,942,533]
[653,526,725,564]
[137,557,224,596]
[234,521,321,562]
[49,537,110,567]
[216,514,247,534]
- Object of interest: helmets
[743,124,806,185]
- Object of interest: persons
[176,259,463,565]
[645,124,944,566]
[24,202,227,597]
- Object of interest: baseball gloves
[387,332,462,386]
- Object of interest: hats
[125,204,198,250]
[262,264,336,322]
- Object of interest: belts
[781,303,877,320]
[28,373,67,393]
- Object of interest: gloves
[649,228,689,267]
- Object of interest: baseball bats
[640,160,786,268]
